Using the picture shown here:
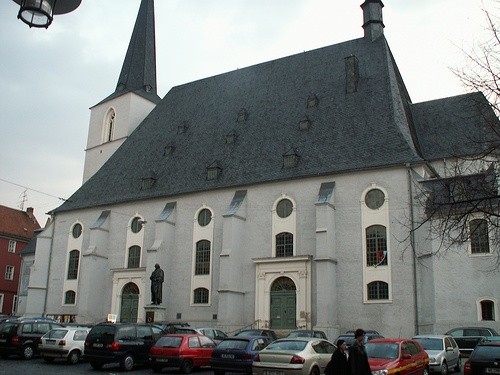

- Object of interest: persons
[150,264,164,305]
[331,339,351,375]
[347,329,372,375]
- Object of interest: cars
[38,327,93,365]
[169,322,387,346]
[210,337,271,375]
[409,334,462,375]
[363,338,411,375]
[252,337,337,375]
[150,334,218,375]
[0,317,65,360]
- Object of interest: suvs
[82,322,165,370]
[443,327,500,358]
